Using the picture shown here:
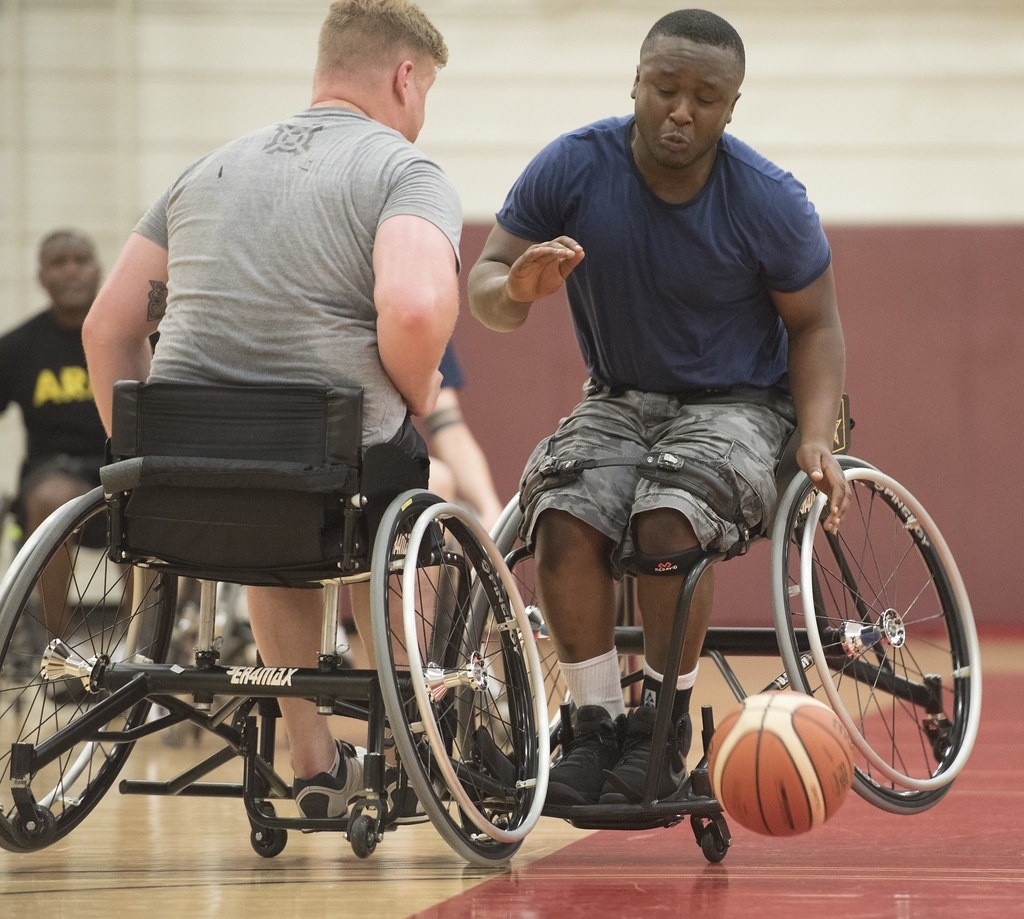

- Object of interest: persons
[468,10,846,807]
[1,227,159,704]
[82,0,505,824]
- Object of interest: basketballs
[705,689,856,839]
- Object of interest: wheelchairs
[0,379,550,871]
[458,393,982,866]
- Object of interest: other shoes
[547,705,693,805]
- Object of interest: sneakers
[294,737,368,834]
